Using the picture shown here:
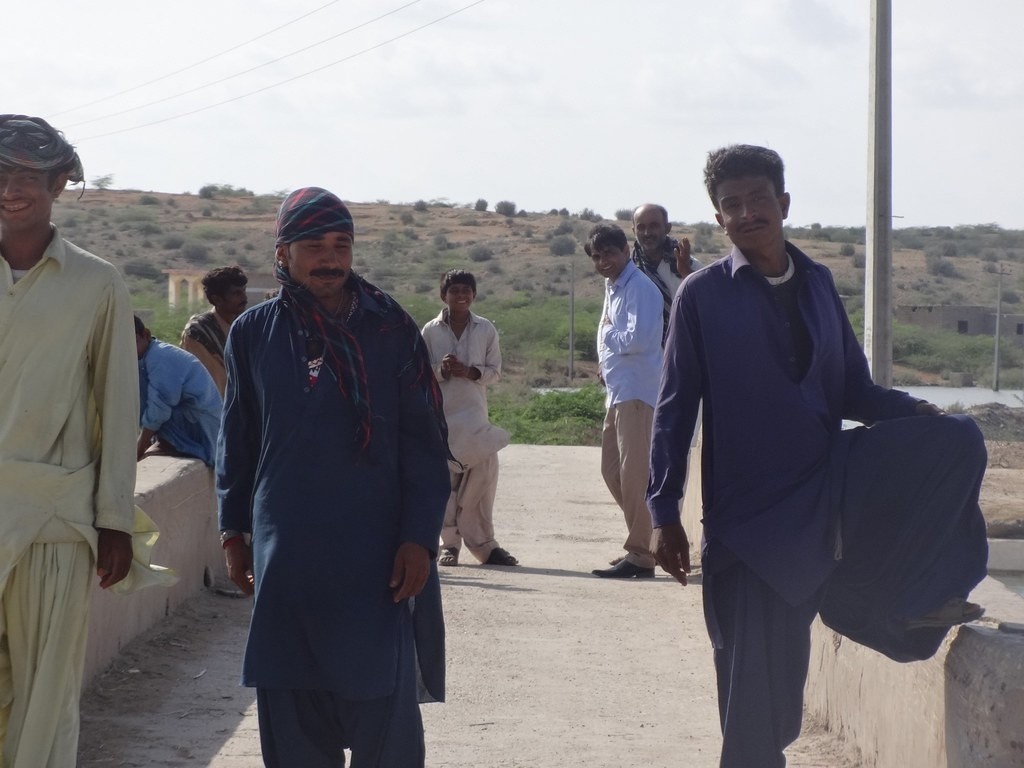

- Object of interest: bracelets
[464,367,470,377]
[223,534,244,551]
[217,529,242,549]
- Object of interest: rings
[446,362,450,365]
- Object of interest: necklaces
[446,313,470,328]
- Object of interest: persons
[178,266,251,406]
[646,144,991,768]
[582,224,667,581]
[418,270,521,568]
[0,114,184,768]
[630,204,705,352]
[135,314,225,470]
[215,187,464,768]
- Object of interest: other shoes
[592,559,655,578]
[896,594,985,633]
[483,547,518,566]
[439,546,459,566]
